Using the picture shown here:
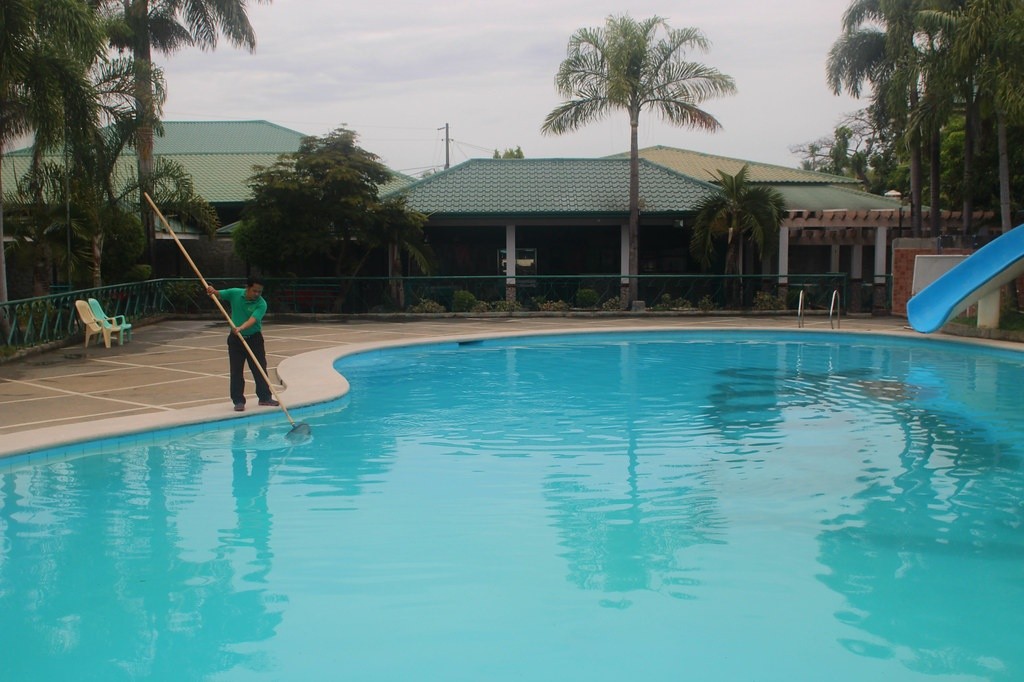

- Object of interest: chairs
[88,298,132,346]
[75,299,121,348]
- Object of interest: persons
[206,276,280,411]
